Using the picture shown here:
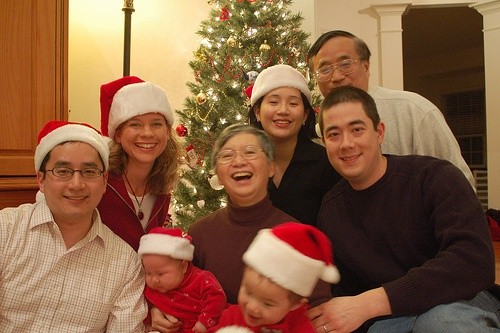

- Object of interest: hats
[137,226,194,261]
[99,75,173,139]
[242,222,340,298]
[247,64,312,106]
[34,120,111,175]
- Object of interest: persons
[138,228,227,333]
[245,64,341,226]
[0,120,148,333]
[97,77,181,253]
[186,123,333,307]
[205,222,340,333]
[308,86,500,333]
[307,30,477,192]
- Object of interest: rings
[324,326,328,332]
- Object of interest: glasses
[215,146,264,164]
[313,58,360,82]
[45,167,104,179]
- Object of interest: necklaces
[123,170,146,220]
